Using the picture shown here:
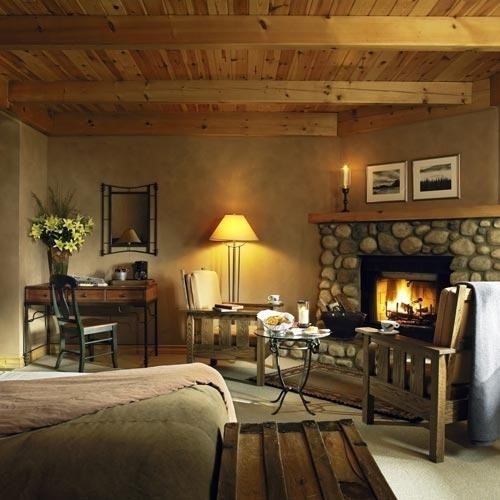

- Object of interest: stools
[215,419,396,500]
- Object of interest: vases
[48,248,69,276]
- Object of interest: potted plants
[115,265,129,282]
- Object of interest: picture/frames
[365,153,461,204]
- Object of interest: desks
[255,325,333,415]
[24,282,158,367]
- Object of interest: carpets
[245,361,425,423]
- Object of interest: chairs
[178,265,284,385]
[49,274,119,372]
[355,281,500,463]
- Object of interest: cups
[268,295,280,302]
[380,320,400,332]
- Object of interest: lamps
[114,228,142,263]
[209,212,259,303]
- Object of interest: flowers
[26,181,94,257]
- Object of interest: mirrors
[101,182,158,257]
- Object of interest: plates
[268,301,283,305]
[379,328,400,335]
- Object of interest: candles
[343,165,348,188]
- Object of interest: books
[212,304,243,313]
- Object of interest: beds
[1,363,239,500]
